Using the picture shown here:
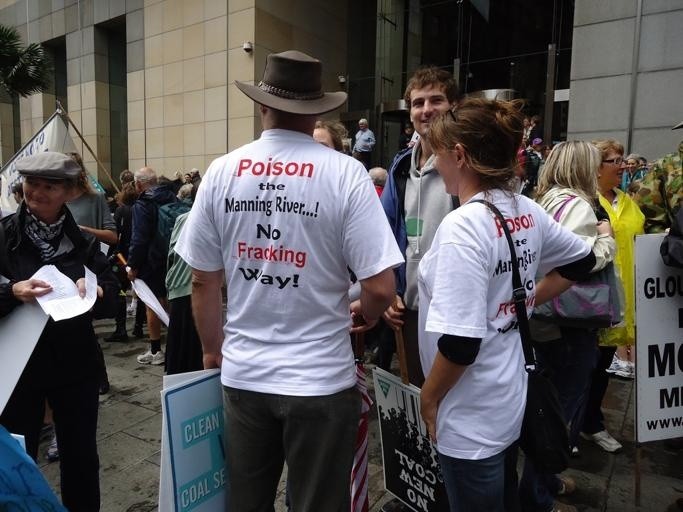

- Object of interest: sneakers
[551,501,576,512]
[557,479,575,494]
[104,322,165,365]
[606,360,635,379]
[581,428,622,452]
[44,437,59,460]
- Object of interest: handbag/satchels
[519,373,570,473]
[552,283,614,328]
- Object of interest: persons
[69,152,225,375]
[520,142,618,510]
[316,114,392,368]
[0,154,120,508]
[518,116,647,454]
[12,185,24,213]
[171,49,406,511]
[379,64,463,508]
[413,94,596,508]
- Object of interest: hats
[17,153,82,182]
[234,50,348,115]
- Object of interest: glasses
[603,157,622,166]
[449,105,457,121]
